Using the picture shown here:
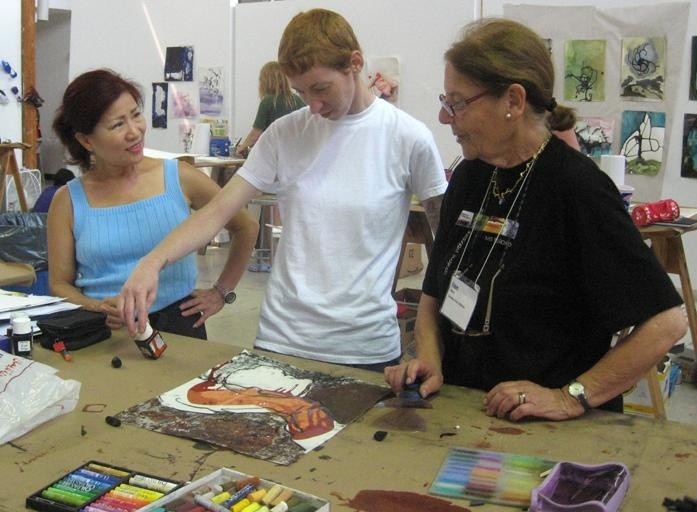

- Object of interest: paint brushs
[235,137,243,146]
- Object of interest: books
[650,215,696,229]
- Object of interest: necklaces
[484,133,551,204]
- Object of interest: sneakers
[248,262,271,272]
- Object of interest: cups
[615,184,635,210]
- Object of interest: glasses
[439,81,511,117]
[451,264,506,337]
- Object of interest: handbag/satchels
[631,199,680,227]
[36,308,111,362]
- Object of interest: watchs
[564,377,593,415]
[212,282,236,305]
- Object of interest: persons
[113,10,459,371]
[28,168,75,214]
[46,67,260,341]
[384,16,691,425]
[237,60,306,271]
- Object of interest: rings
[516,391,528,404]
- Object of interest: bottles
[132,323,169,362]
[10,316,34,361]
